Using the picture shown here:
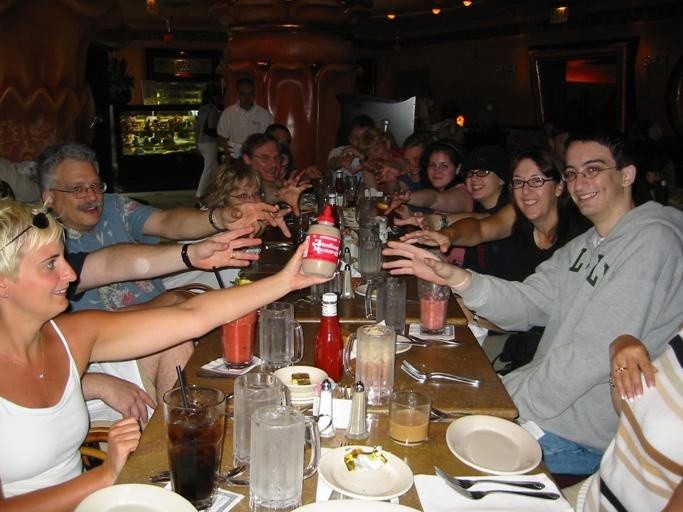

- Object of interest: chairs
[80,426,111,467]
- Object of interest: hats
[462,134,511,185]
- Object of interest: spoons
[148,466,249,487]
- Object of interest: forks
[400,364,479,389]
[402,359,480,383]
[435,467,560,501]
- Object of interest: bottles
[315,378,335,441]
[312,293,345,381]
[347,379,368,441]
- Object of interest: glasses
[226,191,265,201]
[508,177,554,190]
[48,182,108,200]
[464,171,491,179]
[560,166,619,183]
[0,207,56,251]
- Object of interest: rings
[232,251,235,259]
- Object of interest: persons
[0,179,263,433]
[240,133,312,217]
[157,161,293,294]
[216,79,274,167]
[265,124,291,148]
[189,73,224,205]
[0,194,333,512]
[277,145,292,179]
[35,142,277,313]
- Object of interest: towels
[412,471,574,512]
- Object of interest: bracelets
[181,244,193,268]
[209,207,224,231]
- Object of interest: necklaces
[2,335,46,380]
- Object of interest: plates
[292,500,419,511]
[319,445,414,500]
[350,334,414,353]
[201,357,262,377]
[446,414,544,474]
[73,482,198,512]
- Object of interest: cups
[163,386,227,508]
[221,311,258,370]
[363,272,407,336]
[213,170,487,304]
[258,302,304,369]
[232,372,289,473]
[343,324,397,407]
[387,391,430,446]
[417,249,452,335]
[251,405,320,511]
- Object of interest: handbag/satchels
[201,120,220,139]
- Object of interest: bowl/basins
[275,364,329,411]
